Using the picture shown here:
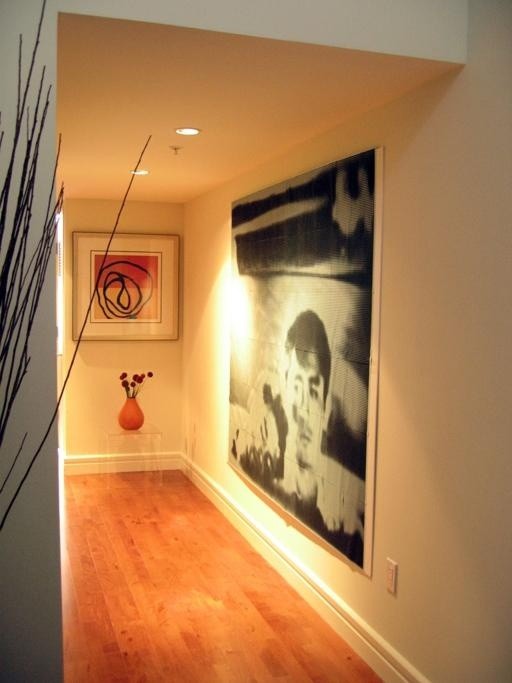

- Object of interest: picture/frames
[228,145,382,578]
[71,230,184,345]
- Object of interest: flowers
[120,370,152,396]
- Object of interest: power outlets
[383,557,397,595]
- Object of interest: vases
[118,393,143,431]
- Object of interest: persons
[229,309,364,568]
